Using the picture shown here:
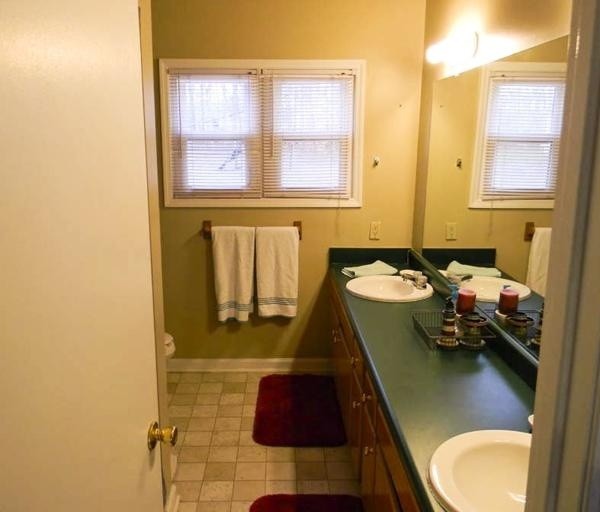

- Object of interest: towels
[253,226,299,319]
[211,224,255,322]
[341,258,399,280]
[445,260,503,280]
[524,225,554,301]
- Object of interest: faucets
[447,274,473,286]
[402,272,428,289]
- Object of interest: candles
[499,288,518,317]
[454,289,477,316]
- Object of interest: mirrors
[420,29,575,365]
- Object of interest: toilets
[164,332,176,358]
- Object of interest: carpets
[251,370,349,447]
[247,493,366,512]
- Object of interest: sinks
[428,430,532,512]
[439,270,531,303]
[345,275,434,303]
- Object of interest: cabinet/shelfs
[327,279,423,512]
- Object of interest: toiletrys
[436,296,459,351]
